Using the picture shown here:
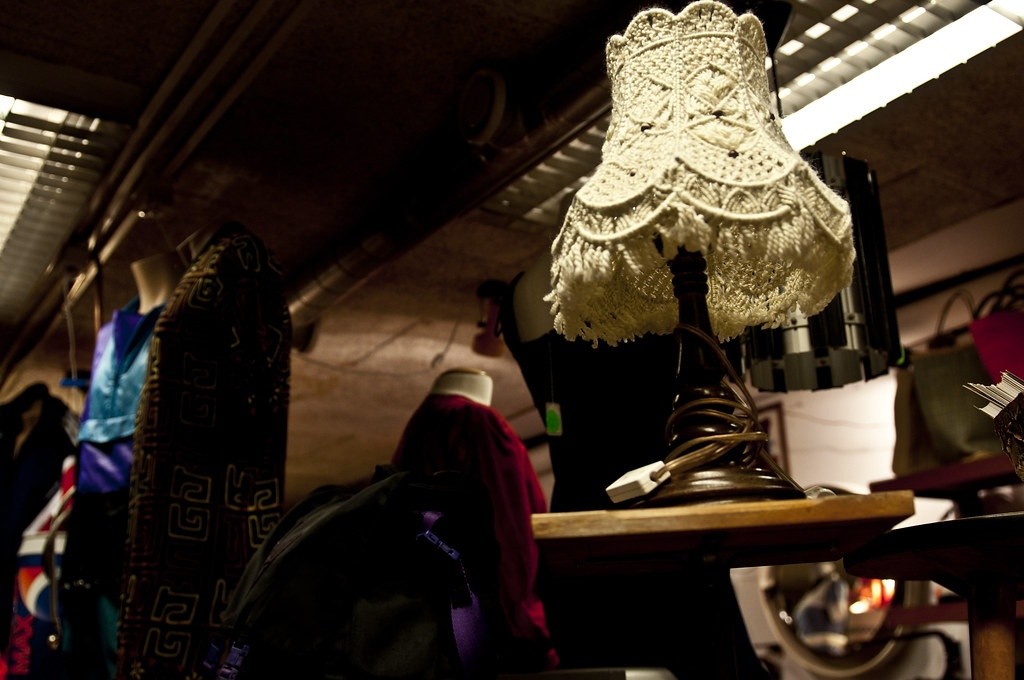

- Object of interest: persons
[76,211,294,680]
[385,366,560,680]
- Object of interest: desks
[530,490,915,680]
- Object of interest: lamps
[543,0,859,507]
